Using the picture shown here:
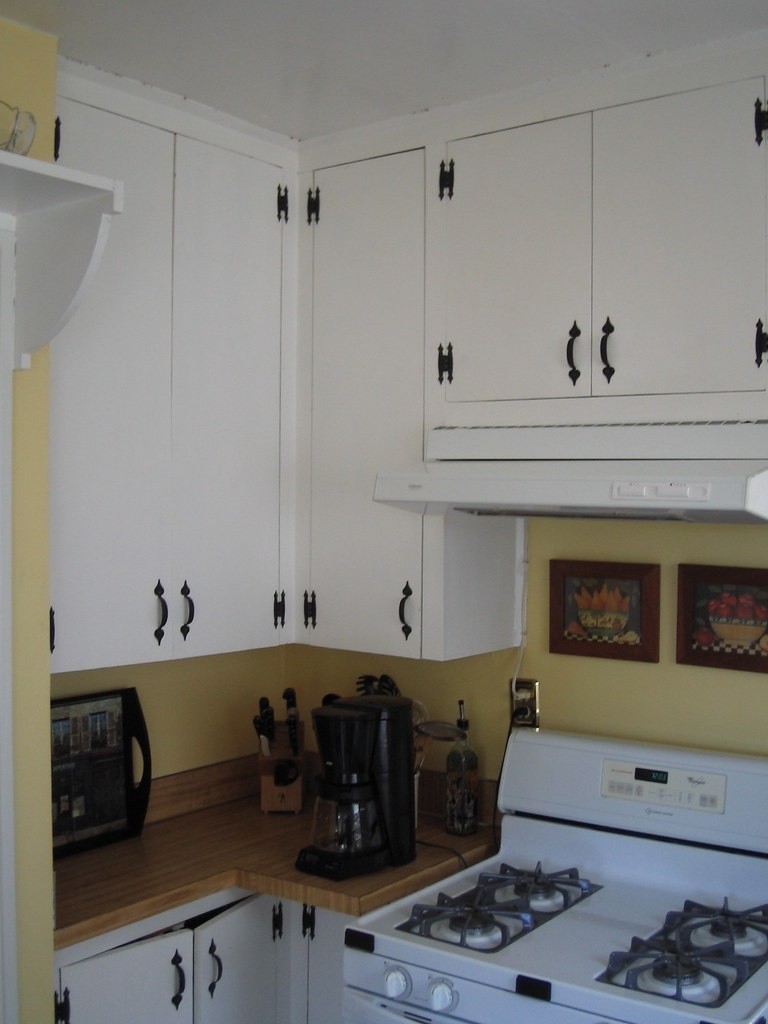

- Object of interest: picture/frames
[548,558,660,664]
[675,562,768,674]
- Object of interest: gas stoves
[342,726,768,1024]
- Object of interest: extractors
[374,459,768,525]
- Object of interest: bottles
[446,719,480,837]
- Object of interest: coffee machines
[294,693,416,882]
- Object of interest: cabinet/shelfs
[302,905,360,1024]
[420,75,767,403]
[47,96,291,672]
[303,145,526,663]
[53,892,284,1024]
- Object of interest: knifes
[251,687,299,755]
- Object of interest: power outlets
[509,677,539,727]
